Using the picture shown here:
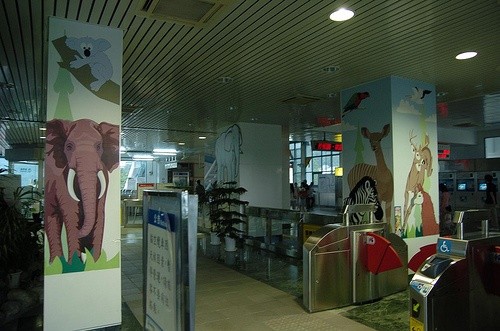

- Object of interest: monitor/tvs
[440,179,453,191]
[456,179,474,192]
[478,179,487,191]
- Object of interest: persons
[195,179,205,199]
[483,175,498,226]
[290,180,315,212]
[440,185,454,235]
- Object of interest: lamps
[311,131,342,152]
[334,167,344,178]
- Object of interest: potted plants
[205,180,250,251]
[1,168,44,284]
